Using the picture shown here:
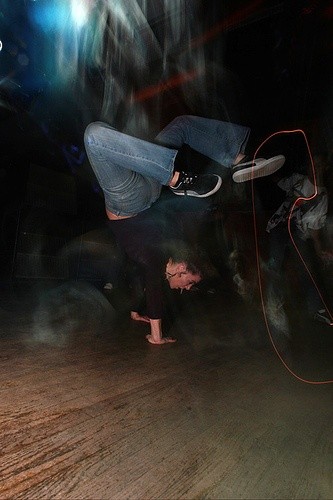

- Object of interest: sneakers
[233,154,285,183]
[172,171,222,198]
[315,309,333,326]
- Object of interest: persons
[82,115,285,344]
[260,148,333,327]
